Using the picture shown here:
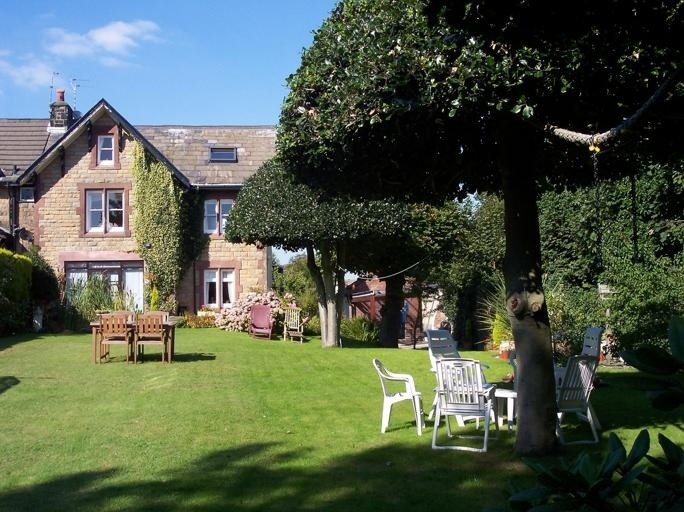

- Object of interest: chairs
[424,327,495,426]
[248,302,275,341]
[370,356,425,438]
[428,356,499,455]
[553,325,606,403]
[282,304,307,345]
[87,310,177,365]
[552,353,605,448]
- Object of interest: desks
[486,385,519,430]
